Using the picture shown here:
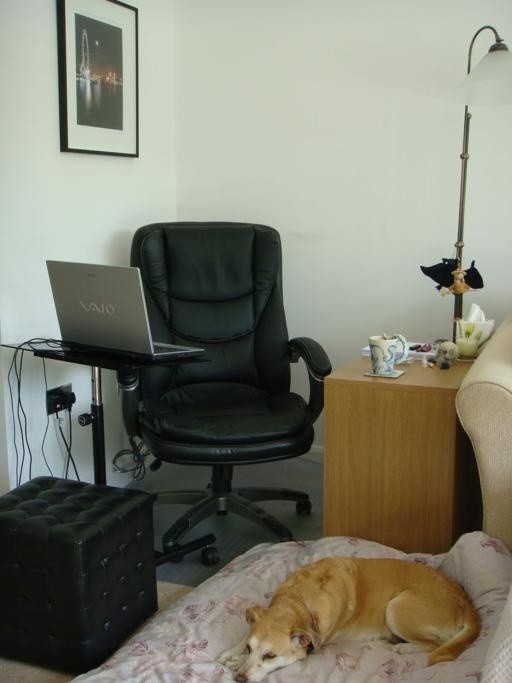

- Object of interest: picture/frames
[57,0,140,157]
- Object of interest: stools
[0,476,158,675]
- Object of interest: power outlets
[43,384,76,418]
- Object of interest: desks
[318,356,475,557]
[1,327,209,485]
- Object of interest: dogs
[233,556,482,682]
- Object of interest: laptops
[46,260,206,357]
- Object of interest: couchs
[455,316,512,556]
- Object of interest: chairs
[125,218,337,562]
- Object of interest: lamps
[440,24,512,345]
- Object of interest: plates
[361,342,438,361]
[362,369,406,379]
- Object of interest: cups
[368,335,409,375]
